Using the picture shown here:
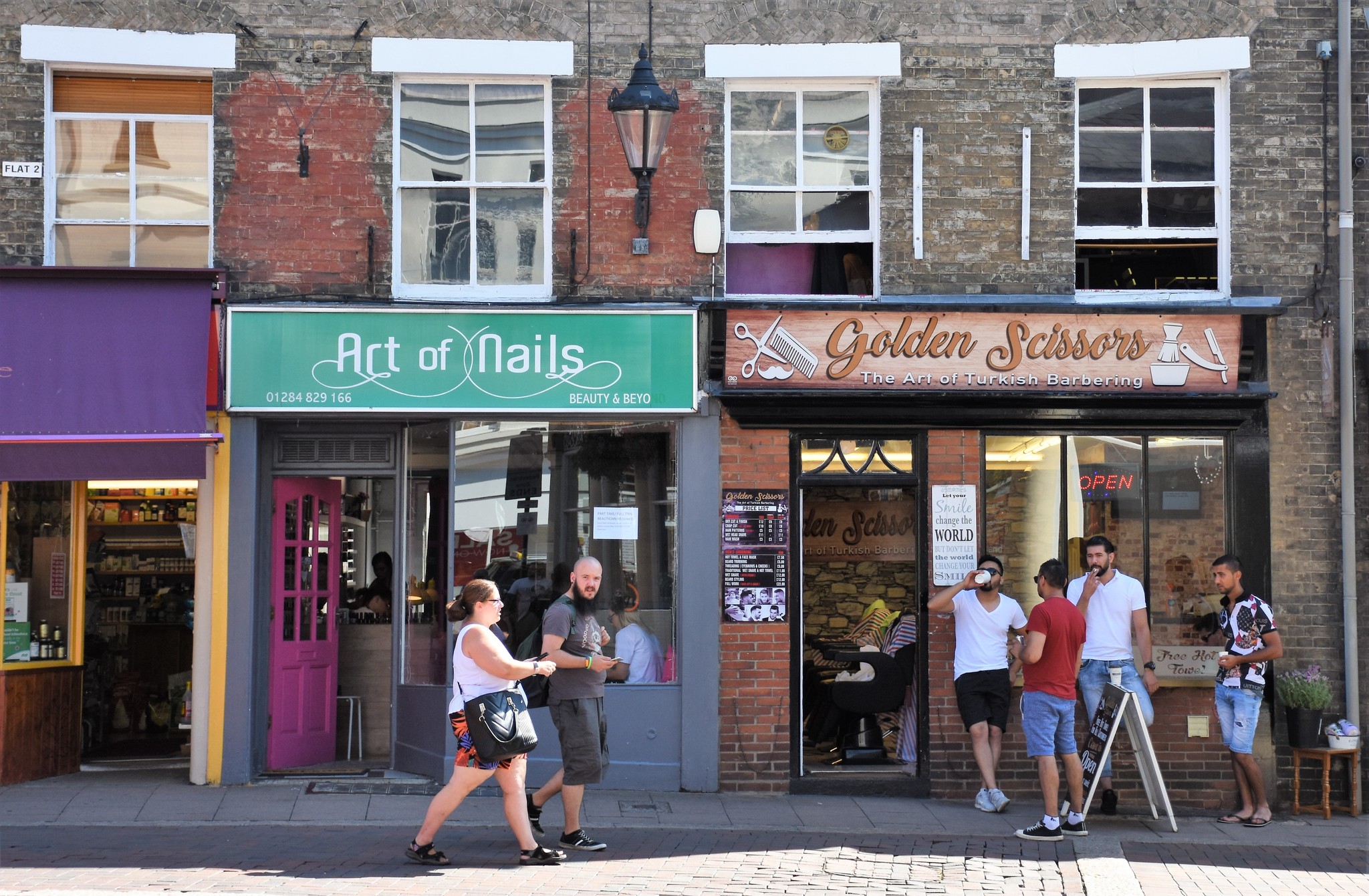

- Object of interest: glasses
[1033,575,1046,583]
[607,611,617,623]
[976,567,1003,577]
[475,597,502,605]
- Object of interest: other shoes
[1099,792,1119,815]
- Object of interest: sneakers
[975,788,997,812]
[988,788,1011,812]
[558,829,607,851]
[1060,818,1088,836]
[1015,819,1063,841]
[526,793,546,838]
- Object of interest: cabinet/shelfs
[87,496,196,625]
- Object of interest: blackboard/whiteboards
[1059,682,1178,832]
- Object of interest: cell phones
[609,657,623,661]
[533,652,548,661]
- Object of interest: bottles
[98,577,125,597]
[179,681,191,725]
[30,619,66,661]
[1337,719,1358,736]
[1167,591,1175,618]
[340,611,430,625]
[139,499,186,522]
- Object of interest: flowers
[355,490,370,505]
[1276,665,1333,708]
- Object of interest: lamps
[608,43,680,238]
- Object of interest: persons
[606,587,664,684]
[1211,553,1283,827]
[926,553,1029,812]
[453,560,572,643]
[1065,536,1160,815]
[308,551,394,624]
[538,555,618,850]
[1007,558,1089,841]
[405,579,568,866]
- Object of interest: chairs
[804,598,915,766]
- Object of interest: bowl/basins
[1327,734,1359,749]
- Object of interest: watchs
[533,660,540,678]
[1143,661,1157,671]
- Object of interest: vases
[1286,706,1322,748]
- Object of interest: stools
[1291,744,1361,820]
[336,696,362,760]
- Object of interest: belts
[1250,689,1265,698]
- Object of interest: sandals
[519,844,567,866]
[404,838,451,865]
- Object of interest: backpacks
[516,597,576,697]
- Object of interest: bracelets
[584,655,592,670]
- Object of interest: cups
[1326,723,1345,736]
[1219,651,1228,662]
[1108,664,1122,684]
[975,570,991,584]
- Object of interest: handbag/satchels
[464,688,538,763]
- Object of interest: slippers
[1244,817,1272,827]
[1216,814,1251,823]
